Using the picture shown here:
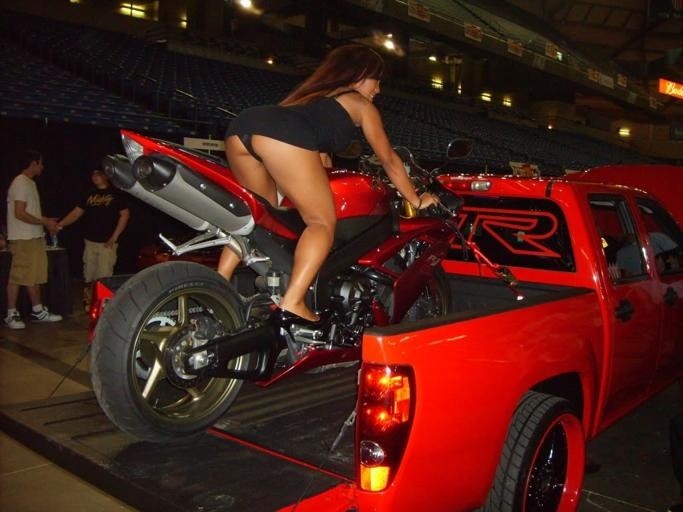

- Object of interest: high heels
[271,307,328,343]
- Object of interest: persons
[51,162,129,325]
[5,149,63,329]
[215,43,439,324]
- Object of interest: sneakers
[29,307,62,324]
[2,311,25,329]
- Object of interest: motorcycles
[83,127,535,446]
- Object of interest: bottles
[50,233,59,249]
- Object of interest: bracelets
[416,196,423,211]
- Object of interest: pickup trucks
[0,158,681,511]
